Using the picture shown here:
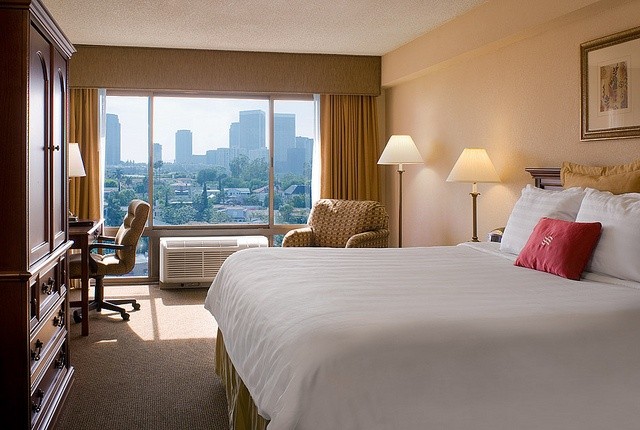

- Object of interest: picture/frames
[580,25,640,142]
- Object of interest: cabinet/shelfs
[0,0,78,272]
[0,240,75,430]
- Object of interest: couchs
[282,199,390,248]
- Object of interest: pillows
[560,161,640,195]
[513,217,602,280]
[204,167,639,430]
[499,183,584,256]
[575,187,640,282]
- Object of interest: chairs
[69,199,150,323]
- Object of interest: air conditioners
[159,235,268,289]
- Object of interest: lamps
[446,149,501,243]
[68,142,86,220]
[377,135,426,248]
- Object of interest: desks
[69,218,105,337]
